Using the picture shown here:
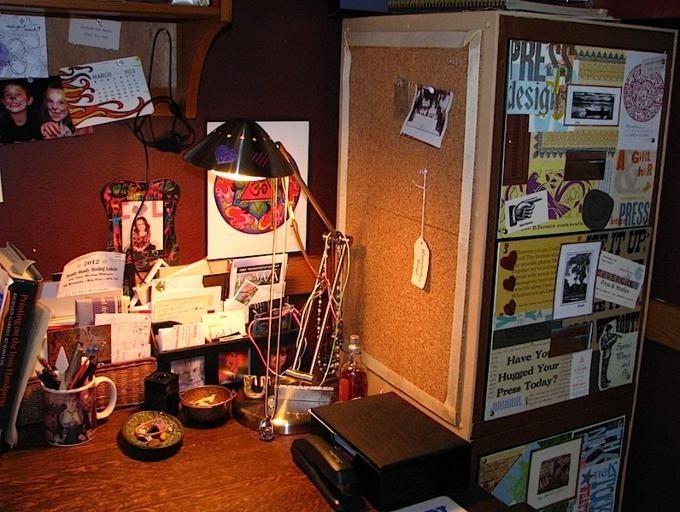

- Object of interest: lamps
[183,117,352,435]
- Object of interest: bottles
[339,333,367,399]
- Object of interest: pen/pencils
[35,341,98,390]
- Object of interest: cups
[40,376,118,447]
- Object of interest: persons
[40,79,73,139]
[173,355,204,395]
[265,346,290,376]
[1,83,35,143]
[508,196,542,228]
[127,218,156,253]
[599,324,619,390]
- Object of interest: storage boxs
[311,392,472,512]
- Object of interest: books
[1,242,45,448]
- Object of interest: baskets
[16,355,160,430]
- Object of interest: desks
[0,388,510,512]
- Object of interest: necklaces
[316,232,347,377]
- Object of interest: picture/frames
[204,119,310,262]
[102,179,180,274]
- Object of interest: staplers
[291,434,366,512]
